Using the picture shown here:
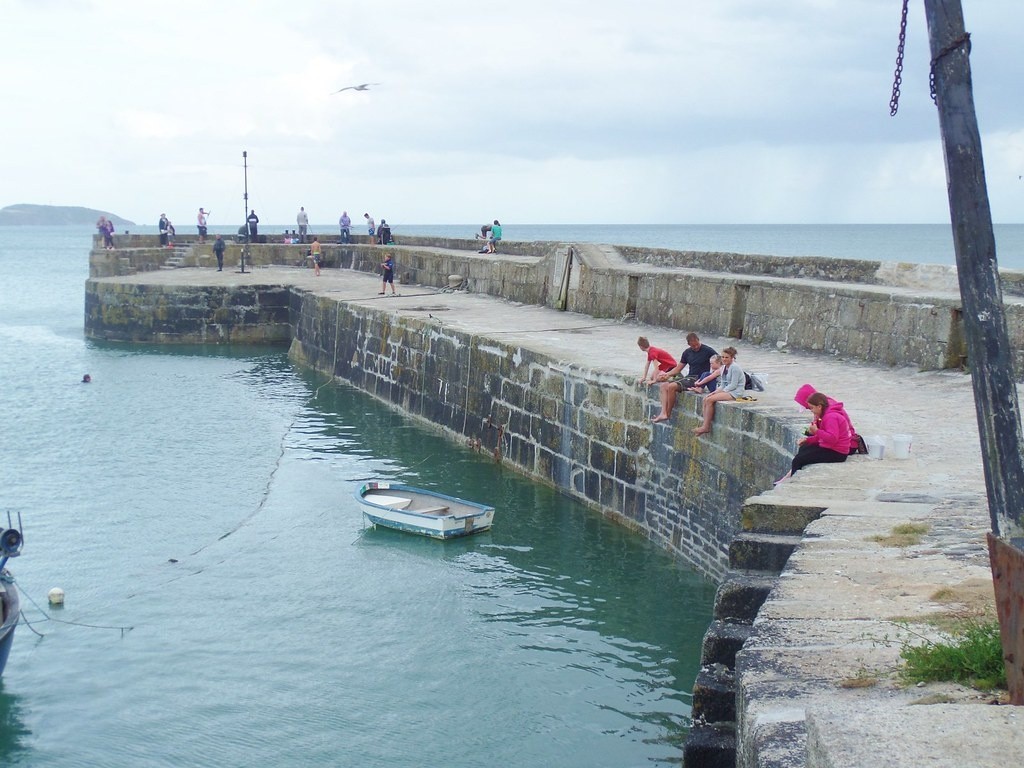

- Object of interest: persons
[651,333,718,423]
[378,254,395,294]
[96,214,115,250]
[339,211,351,243]
[159,214,175,248]
[197,207,208,244]
[82,374,91,382]
[238,224,247,234]
[212,234,225,271]
[771,392,851,485]
[687,355,723,394]
[247,210,259,243]
[795,384,859,455]
[637,336,683,385]
[311,237,321,275]
[475,223,500,239]
[364,213,375,244]
[487,220,502,253]
[377,220,389,244]
[297,207,308,243]
[691,346,746,436]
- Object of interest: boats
[0,511,26,677]
[354,480,497,540]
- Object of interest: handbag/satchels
[743,370,752,390]
[855,435,868,455]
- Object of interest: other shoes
[378,292,384,295]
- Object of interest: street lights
[242,150,253,266]
